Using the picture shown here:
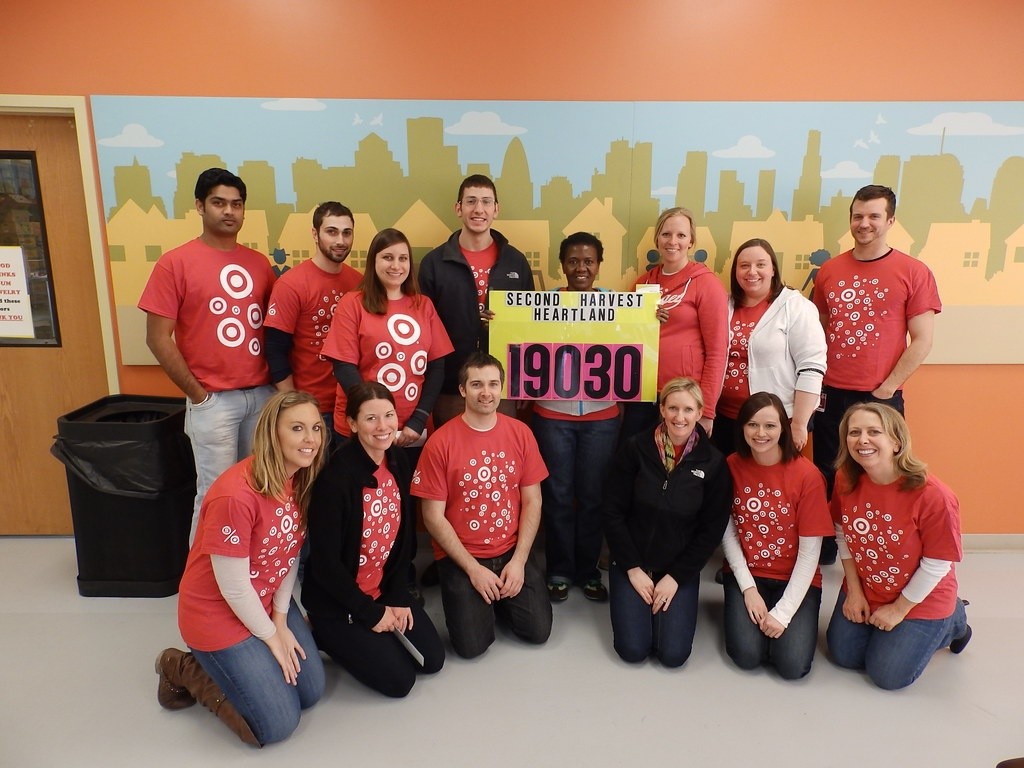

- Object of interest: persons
[155,391,327,748]
[300,380,445,698]
[723,392,835,680]
[137,167,942,608]
[409,352,552,659]
[602,377,735,667]
[825,401,972,691]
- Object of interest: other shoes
[599,539,610,571]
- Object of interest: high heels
[950,600,973,653]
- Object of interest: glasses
[458,198,496,206]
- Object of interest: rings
[879,625,884,629]
[660,599,666,602]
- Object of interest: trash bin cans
[57,392,199,598]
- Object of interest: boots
[155,648,262,749]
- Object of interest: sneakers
[547,582,568,602]
[572,577,608,601]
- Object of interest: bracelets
[193,393,208,406]
